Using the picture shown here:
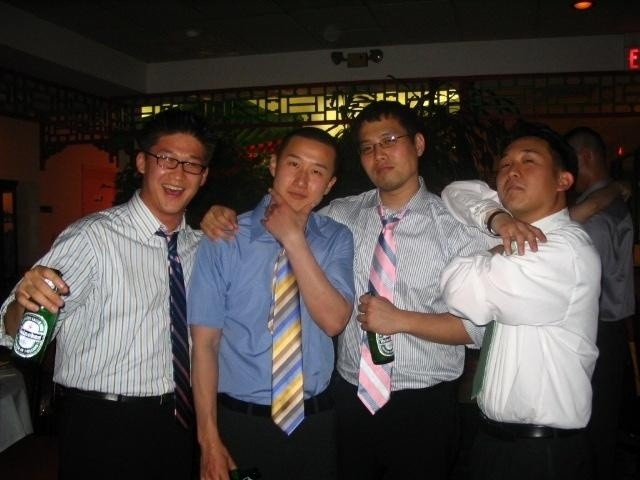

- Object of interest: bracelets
[487,210,512,236]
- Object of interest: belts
[219,391,334,417]
[55,384,175,406]
[484,421,572,438]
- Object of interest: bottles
[11,266,64,373]
[360,290,395,364]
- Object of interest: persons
[200,100,488,480]
[441,121,602,479]
[1,108,240,480]
[567,176,633,224]
[565,124,637,358]
[187,126,355,479]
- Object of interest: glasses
[146,152,207,175]
[358,134,407,155]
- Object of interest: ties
[470,240,517,400]
[270,247,305,436]
[357,202,408,416]
[154,229,196,429]
